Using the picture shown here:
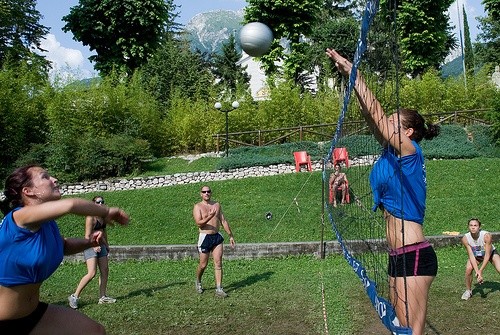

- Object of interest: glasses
[201,190,211,193]
[96,200,104,204]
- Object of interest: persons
[68,196,118,309]
[192,185,235,297]
[459,219,500,300]
[323,47,440,335]
[330,164,348,208]
[0,166,128,335]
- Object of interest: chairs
[293,151,312,172]
[329,178,349,205]
[333,148,348,170]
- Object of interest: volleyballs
[238,22,273,56]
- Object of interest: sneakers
[98,295,117,304]
[195,280,203,294]
[68,294,81,309]
[214,288,228,298]
[461,289,473,301]
